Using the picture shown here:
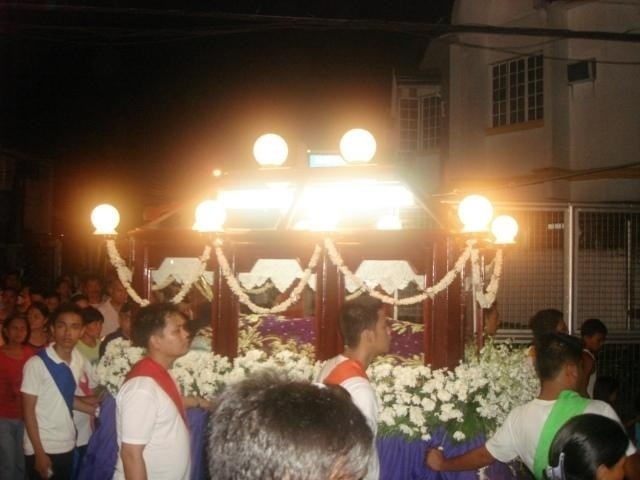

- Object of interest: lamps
[254,133,286,166]
[339,127,377,168]
[492,216,517,245]
[458,195,492,232]
[374,176,412,217]
[91,203,121,234]
[191,200,224,231]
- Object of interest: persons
[107,300,196,480]
[542,413,629,480]
[73,304,103,370]
[95,275,129,342]
[481,300,501,341]
[311,291,393,480]
[0,313,38,480]
[16,300,100,479]
[99,302,141,357]
[524,308,569,364]
[80,274,104,308]
[24,302,53,354]
[69,295,90,311]
[0,287,17,318]
[578,317,607,400]
[424,333,640,480]
[206,376,373,479]
[0,266,89,317]
